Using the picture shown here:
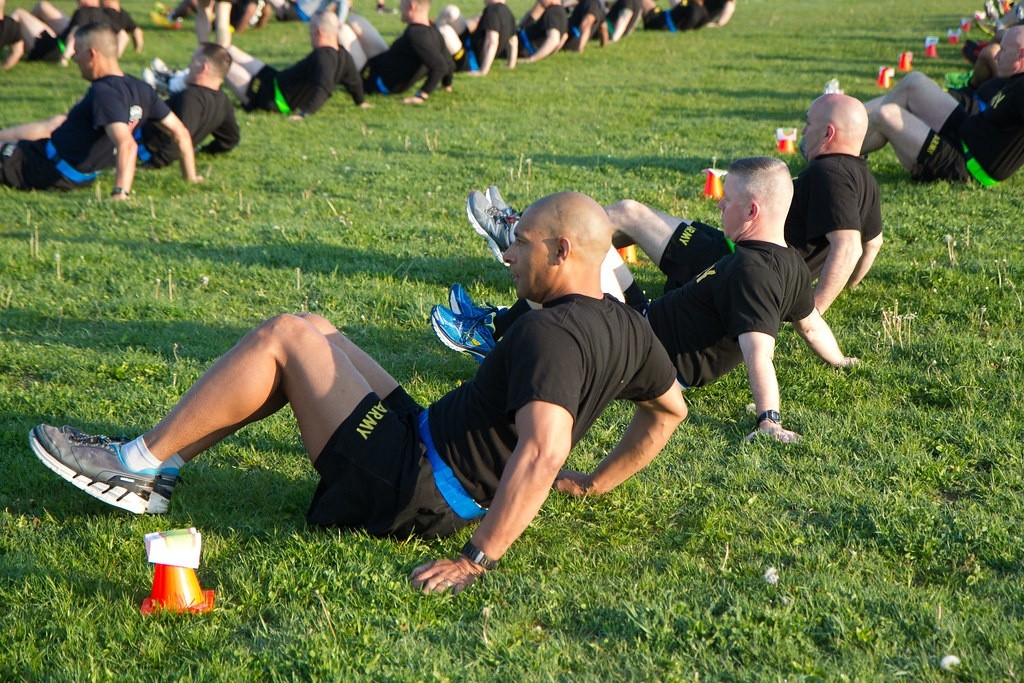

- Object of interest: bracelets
[61,56,69,61]
[288,111,305,117]
[715,21,721,28]
[414,92,426,101]
[111,187,129,196]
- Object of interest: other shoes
[151,1,182,29]
[984,0,1015,22]
[825,77,841,98]
[962,40,980,64]
[150,56,190,79]
[971,19,997,37]
[142,67,183,96]
[378,7,399,14]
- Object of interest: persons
[429,158,860,446]
[798,0,1024,187]
[464,95,884,318]
[0,0,735,202]
[27,190,688,594]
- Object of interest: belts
[465,37,480,71]
[418,407,488,521]
[665,8,678,33]
[607,20,614,39]
[520,28,535,55]
[136,126,151,163]
[273,77,293,113]
[293,2,313,21]
[376,74,392,94]
[46,139,98,186]
[57,38,74,64]
[960,139,999,187]
[568,17,581,39]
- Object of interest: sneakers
[27,422,178,515]
[430,282,509,368]
[466,183,521,268]
[944,68,973,88]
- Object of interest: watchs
[755,410,781,430]
[460,540,500,570]
